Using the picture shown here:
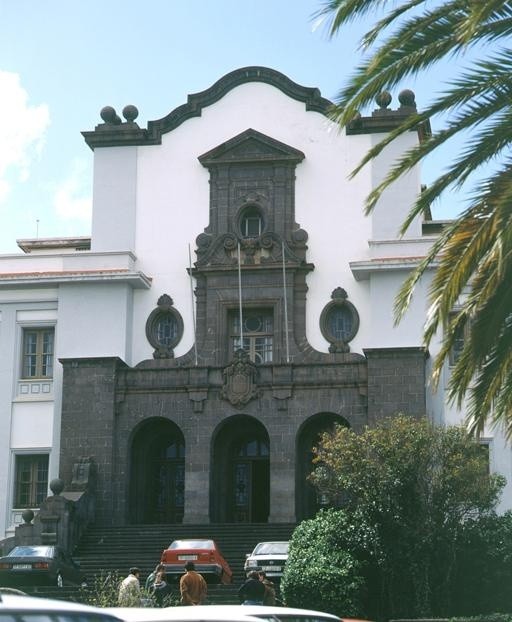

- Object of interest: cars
[243,540,296,587]
[158,538,234,590]
[1,543,87,597]
[0,587,357,621]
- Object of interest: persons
[118,566,153,607]
[145,563,168,590]
[237,570,265,604]
[56,568,64,587]
[149,572,173,608]
[257,570,277,606]
[179,560,208,605]
